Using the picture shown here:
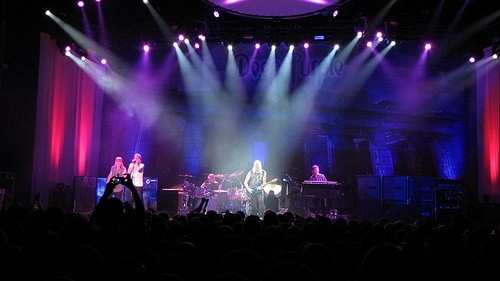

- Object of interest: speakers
[157,189,183,213]
[357,174,435,223]
[73,176,97,214]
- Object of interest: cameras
[115,177,124,184]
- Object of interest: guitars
[246,178,279,198]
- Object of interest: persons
[0,173,500,281]
[106,157,126,199]
[309,165,327,216]
[244,160,266,220]
[128,153,145,209]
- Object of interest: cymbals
[178,174,195,178]
[230,170,244,178]
[213,174,231,177]
